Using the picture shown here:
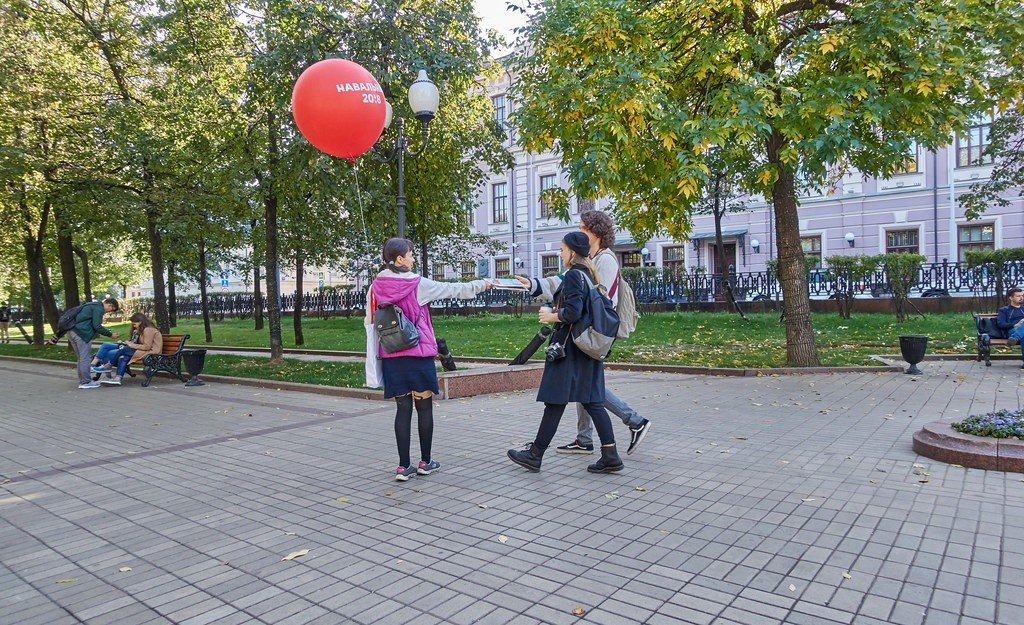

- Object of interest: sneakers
[91,365,112,373]
[626,417,652,455]
[417,459,441,475]
[100,379,121,387]
[556,438,595,454]
[395,464,418,481]
[78,379,101,389]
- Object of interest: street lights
[373,69,440,239]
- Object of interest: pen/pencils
[899,336,929,374]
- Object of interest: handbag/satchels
[979,317,1004,338]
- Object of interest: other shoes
[1007,337,1017,346]
[96,375,114,384]
[90,365,97,373]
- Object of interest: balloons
[292,59,386,163]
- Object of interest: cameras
[544,342,567,362]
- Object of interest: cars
[744,269,872,300]
[10,307,29,320]
[908,260,1024,299]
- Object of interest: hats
[564,231,590,258]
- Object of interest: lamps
[845,233,854,248]
[515,257,524,268]
[642,248,650,261]
[750,239,759,253]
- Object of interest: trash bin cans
[181,349,206,385]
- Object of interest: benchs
[93,334,192,386]
[975,313,1022,367]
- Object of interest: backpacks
[594,251,641,339]
[1,308,10,321]
[560,269,620,362]
[57,301,101,330]
[373,301,418,354]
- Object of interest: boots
[507,439,548,472]
[587,443,625,473]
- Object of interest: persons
[365,238,494,481]
[515,212,650,454]
[0,301,11,344]
[91,314,163,387]
[504,232,624,473]
[68,298,119,388]
[997,288,1024,370]
